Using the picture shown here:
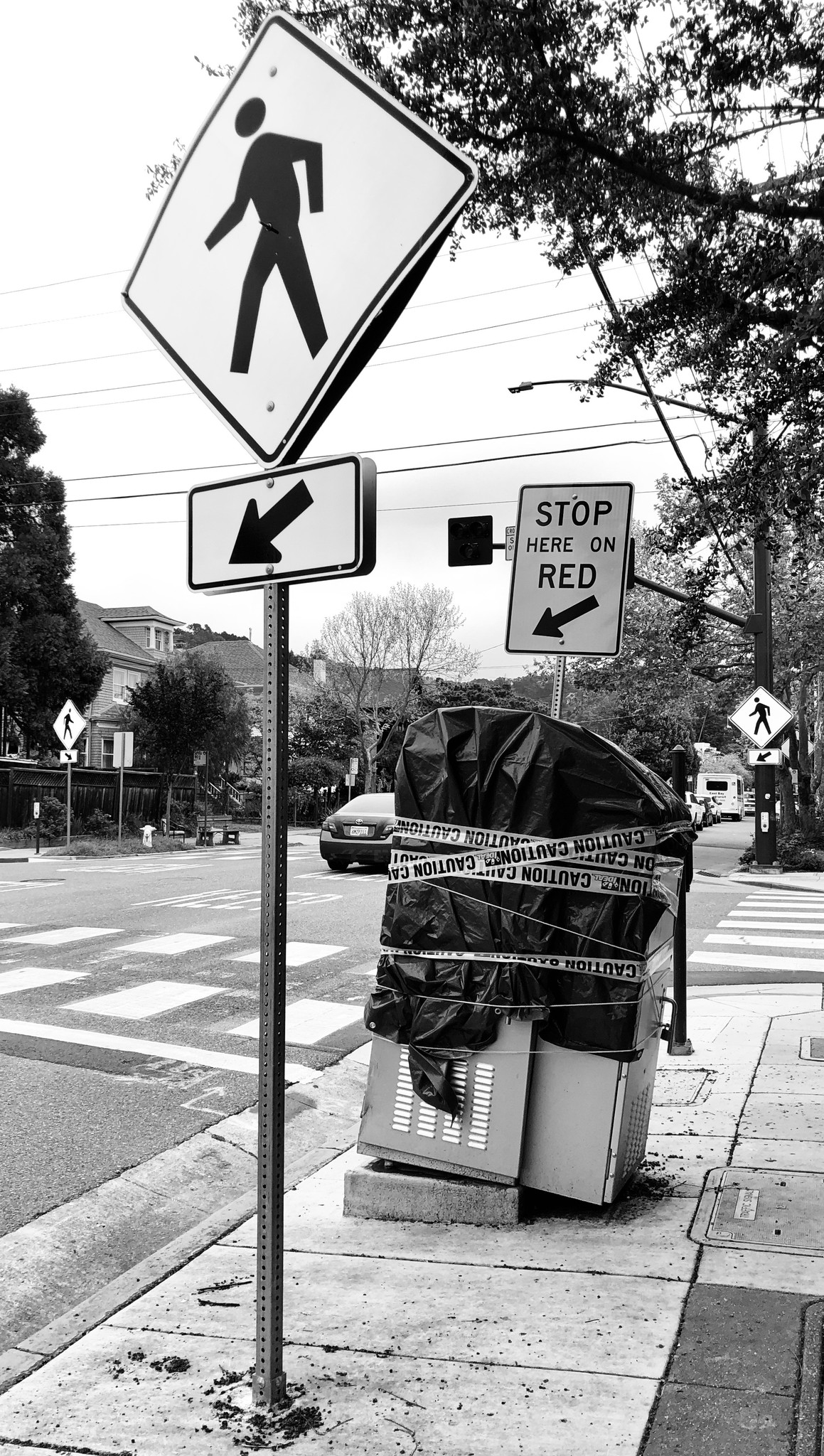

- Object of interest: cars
[695,797,713,827]
[684,791,704,831]
[319,792,395,873]
[744,802,755,816]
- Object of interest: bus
[696,773,745,821]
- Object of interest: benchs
[196,815,240,847]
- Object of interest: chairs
[160,817,186,845]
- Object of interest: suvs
[693,793,722,824]
[743,798,756,803]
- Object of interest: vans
[743,791,756,799]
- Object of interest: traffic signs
[504,480,633,655]
